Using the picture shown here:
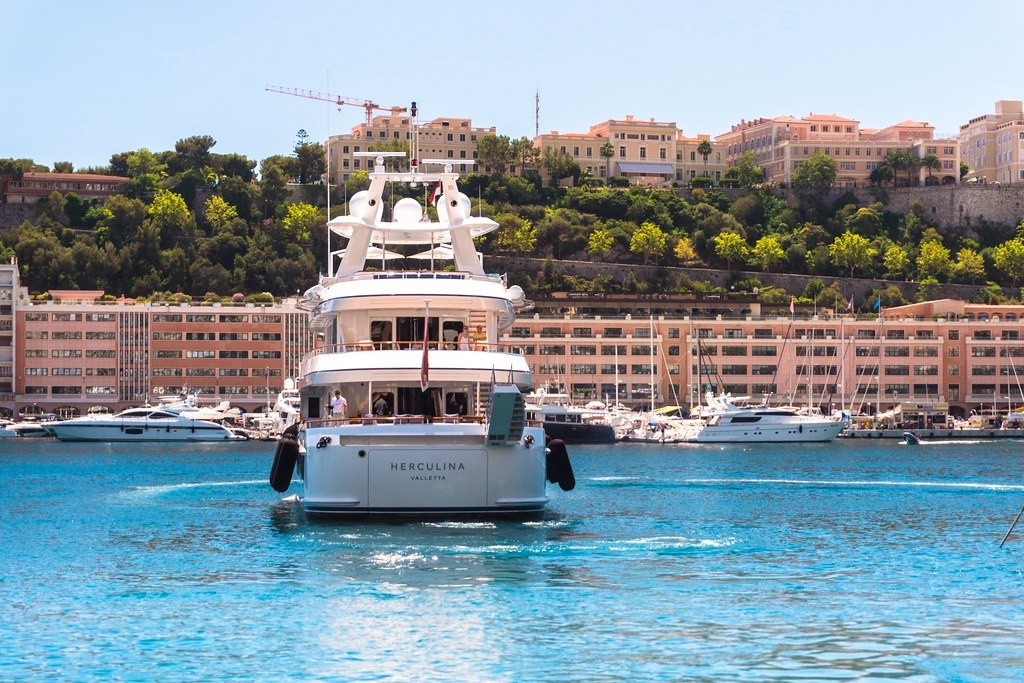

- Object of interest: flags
[428,177,442,207]
[420,299,432,393]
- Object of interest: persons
[374,393,389,416]
[329,390,348,427]
[458,326,470,351]
[473,325,486,341]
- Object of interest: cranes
[265,84,407,127]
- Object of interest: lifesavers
[270,438,300,493]
[547,439,575,491]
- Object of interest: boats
[524,316,850,442]
[902,432,919,445]
[6,421,50,437]
[41,408,248,440]
[272,102,576,521]
